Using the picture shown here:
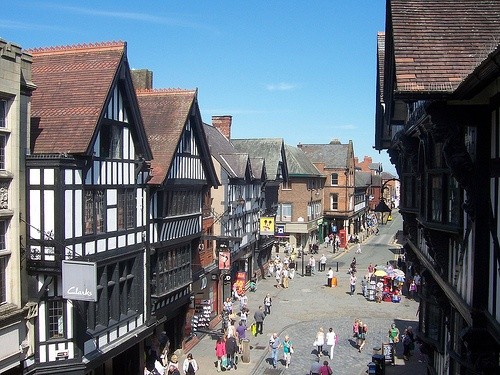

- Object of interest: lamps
[373,178,400,225]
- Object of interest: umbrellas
[373,265,405,280]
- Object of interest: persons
[388,322,414,361]
[254,306,265,336]
[146,330,198,375]
[309,359,332,375]
[361,275,369,297]
[347,256,357,275]
[281,336,293,368]
[326,328,336,360]
[268,240,326,289]
[215,290,249,372]
[263,294,271,316]
[352,318,367,352]
[368,263,378,277]
[407,263,426,301]
[375,280,402,303]
[349,272,358,297]
[327,268,333,287]
[316,327,325,358]
[269,333,281,369]
[325,213,379,253]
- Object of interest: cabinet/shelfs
[190,299,212,336]
[366,273,377,301]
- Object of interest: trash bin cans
[306,265,311,276]
[367,354,385,375]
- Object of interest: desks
[382,342,395,364]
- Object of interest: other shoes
[286,365,288,368]
[231,368,234,370]
[404,356,408,360]
[260,332,263,334]
[359,348,361,353]
[228,365,231,370]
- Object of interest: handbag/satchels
[290,346,294,353]
[327,342,333,345]
[328,367,332,374]
[313,340,316,346]
